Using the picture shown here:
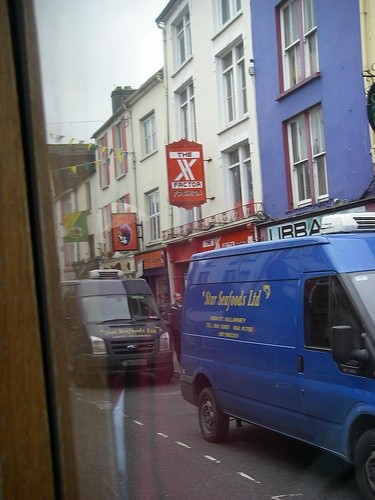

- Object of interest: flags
[63,212,89,242]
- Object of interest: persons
[171,293,183,366]
[160,298,171,340]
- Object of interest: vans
[59,269,175,389]
[180,211,375,500]
